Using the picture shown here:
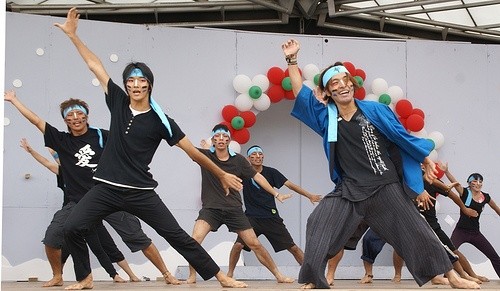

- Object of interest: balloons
[207,61,445,198]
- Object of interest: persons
[53,7,251,290]
[282,38,482,290]
[4,90,500,287]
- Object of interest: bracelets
[285,54,298,65]
